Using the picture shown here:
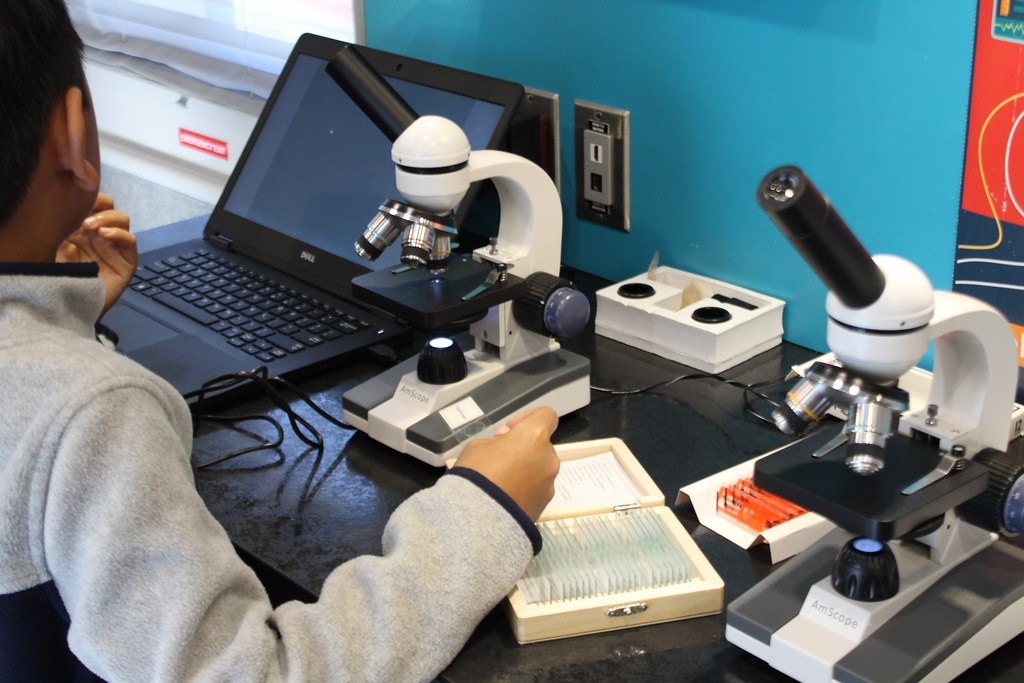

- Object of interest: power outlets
[572,98,632,235]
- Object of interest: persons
[0,0,560,683]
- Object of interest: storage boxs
[595,264,786,374]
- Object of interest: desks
[94,208,1024,683]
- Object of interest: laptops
[96,33,524,407]
